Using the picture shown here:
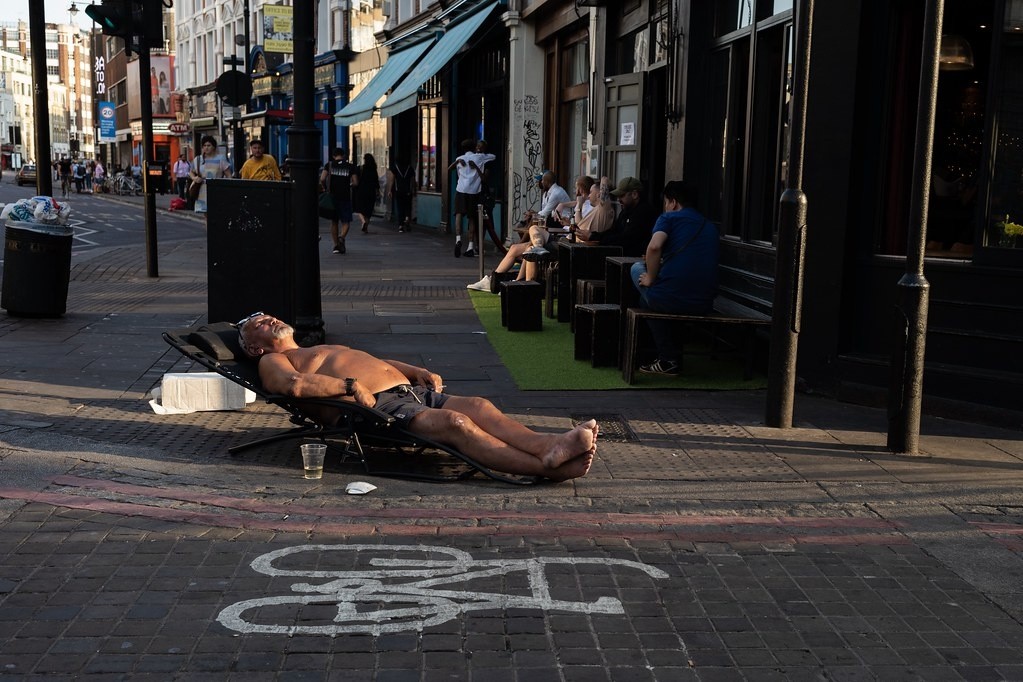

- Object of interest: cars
[16,163,37,186]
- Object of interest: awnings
[333,32,444,126]
[379,0,507,117]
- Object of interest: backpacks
[395,164,411,193]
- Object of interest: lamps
[940,35,974,69]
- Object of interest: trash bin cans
[1,218,74,318]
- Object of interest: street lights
[68,0,99,162]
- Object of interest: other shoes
[337,236,346,254]
[404,220,411,232]
[361,222,368,233]
[463,249,475,257]
[454,241,462,258]
[333,245,339,253]
[398,228,404,233]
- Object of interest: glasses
[232,312,265,350]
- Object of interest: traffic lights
[85,0,144,40]
[217,70,254,107]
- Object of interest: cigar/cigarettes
[431,385,447,390]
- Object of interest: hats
[609,177,643,196]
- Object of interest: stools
[573,302,622,368]
[500,280,542,331]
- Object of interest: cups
[300,444,327,480]
[538,218,546,230]
[533,213,541,226]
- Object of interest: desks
[512,227,569,238]
[556,240,624,332]
[603,253,663,305]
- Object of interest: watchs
[345,377,357,396]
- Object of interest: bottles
[569,215,576,244]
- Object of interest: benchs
[623,307,770,385]
[544,265,559,318]
[576,278,606,304]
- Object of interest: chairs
[163,320,549,482]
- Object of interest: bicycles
[59,173,72,202]
[96,170,146,197]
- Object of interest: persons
[190,135,233,216]
[236,312,599,482]
[319,148,380,253]
[631,180,719,376]
[239,140,289,181]
[489,170,657,281]
[173,154,190,199]
[387,150,417,232]
[151,67,170,113]
[448,138,496,257]
[52,156,141,194]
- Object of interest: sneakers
[522,246,551,262]
[640,358,681,378]
[466,275,491,292]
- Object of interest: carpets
[468,287,771,390]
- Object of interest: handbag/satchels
[169,197,187,211]
[189,155,202,200]
[318,190,336,220]
